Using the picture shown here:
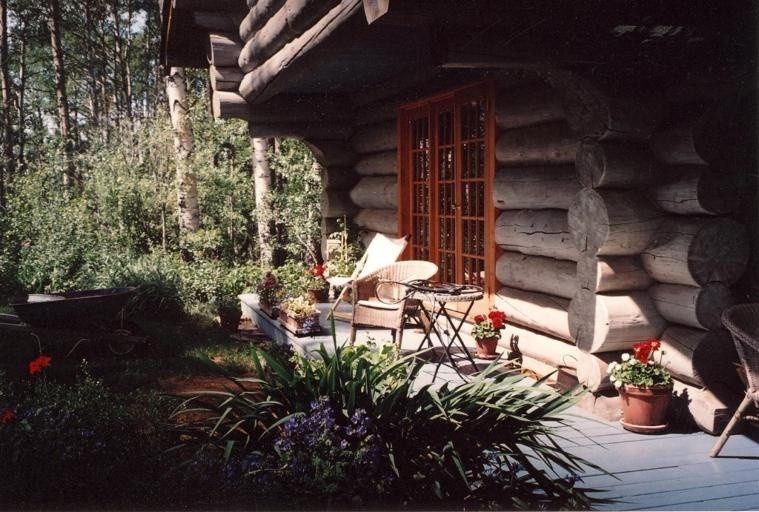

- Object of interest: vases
[307,288,328,305]
[614,383,673,434]
[475,336,501,359]
[258,300,282,317]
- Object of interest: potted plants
[277,298,321,336]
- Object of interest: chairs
[326,233,410,321]
[350,260,439,351]
[711,302,759,458]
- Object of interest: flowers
[305,262,331,288]
[606,337,672,391]
[255,272,285,300]
[471,304,507,340]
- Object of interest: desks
[408,283,483,384]
